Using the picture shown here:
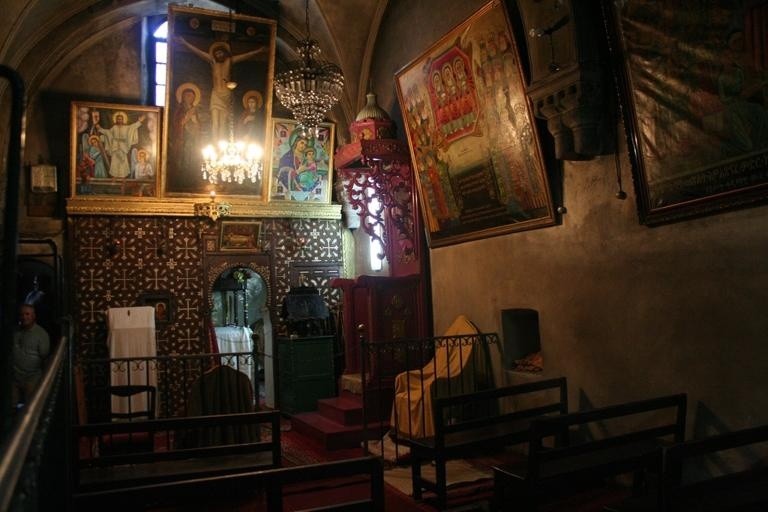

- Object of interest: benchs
[54,374,766,510]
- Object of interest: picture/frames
[139,294,176,326]
[219,220,262,256]
[267,115,337,203]
[68,97,165,200]
[162,3,280,202]
[587,0,768,230]
[392,0,560,253]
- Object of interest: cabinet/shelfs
[274,333,336,422]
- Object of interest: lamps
[272,0,353,145]
[201,0,268,188]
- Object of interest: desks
[214,325,257,405]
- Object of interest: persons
[6,304,50,433]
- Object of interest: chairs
[391,315,495,441]
